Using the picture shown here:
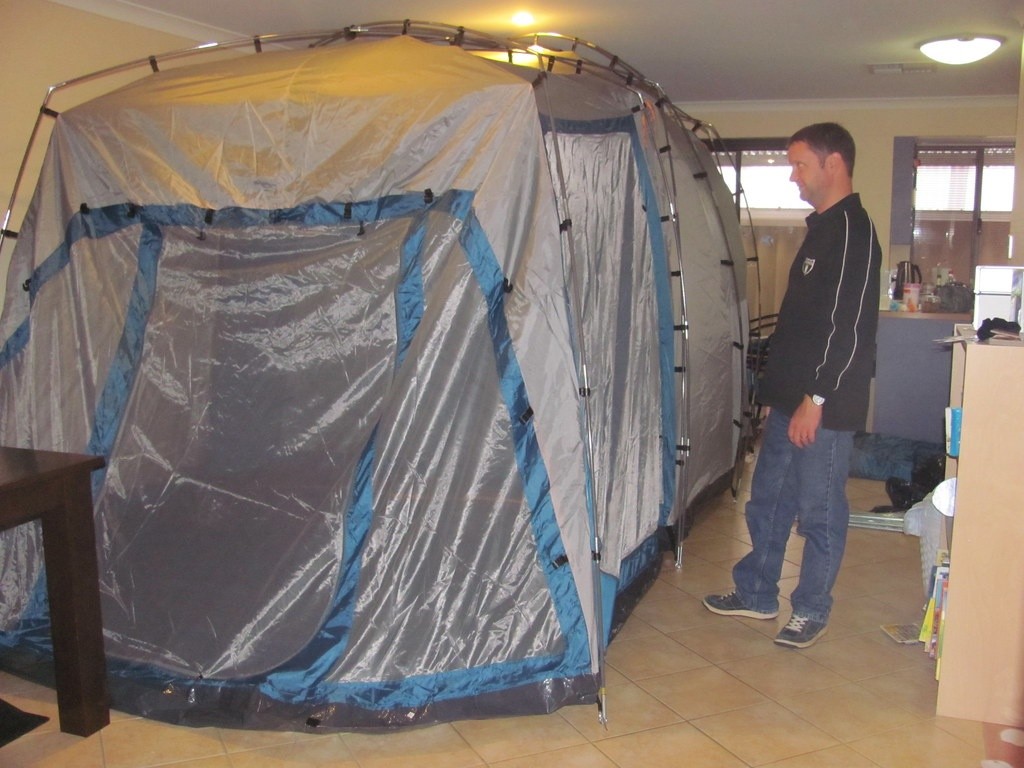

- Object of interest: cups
[903,283,921,311]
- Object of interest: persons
[701,123,882,648]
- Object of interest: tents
[0,33,750,735]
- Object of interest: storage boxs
[974,266,1024,331]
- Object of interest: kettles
[893,261,922,300]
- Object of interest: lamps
[915,32,1007,66]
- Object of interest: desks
[0,441,110,738]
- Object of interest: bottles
[935,274,942,292]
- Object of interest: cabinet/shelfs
[926,324,1024,728]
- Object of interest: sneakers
[701,590,779,620]
[774,613,828,647]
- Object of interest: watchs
[808,393,825,405]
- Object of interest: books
[919,549,951,679]
